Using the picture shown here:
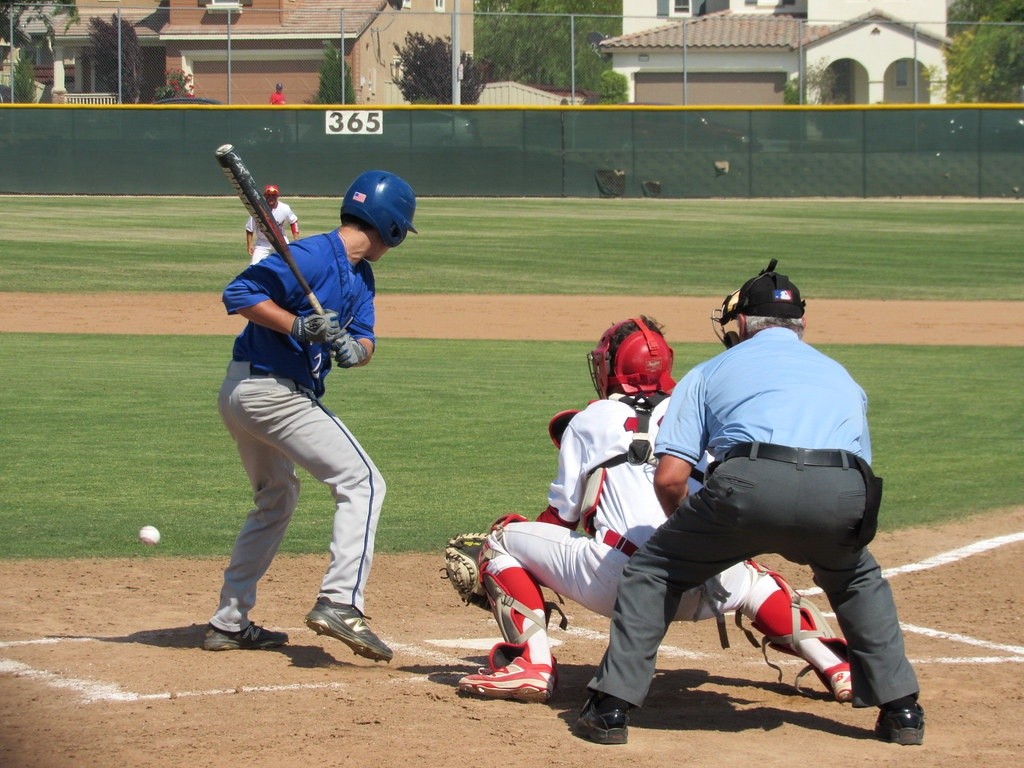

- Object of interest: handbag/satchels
[856,455,884,553]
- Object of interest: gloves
[329,333,368,369]
[291,308,347,344]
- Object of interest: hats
[737,271,806,318]
[262,185,280,197]
[276,83,283,90]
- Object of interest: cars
[156,98,223,104]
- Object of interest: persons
[204,171,419,663]
[573,256,926,746]
[271,82,286,104]
[246,185,299,266]
[459,315,852,703]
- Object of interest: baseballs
[139,525,161,545]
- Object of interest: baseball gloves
[438,531,494,614]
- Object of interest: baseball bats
[213,142,359,370]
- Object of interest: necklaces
[337,230,348,255]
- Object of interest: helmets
[340,169,418,246]
[612,329,677,395]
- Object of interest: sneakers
[823,663,853,703]
[575,692,629,744]
[303,597,393,663]
[458,654,557,702]
[204,621,289,650]
[875,703,924,745]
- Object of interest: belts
[251,365,269,377]
[719,443,859,468]
[605,531,638,558]
